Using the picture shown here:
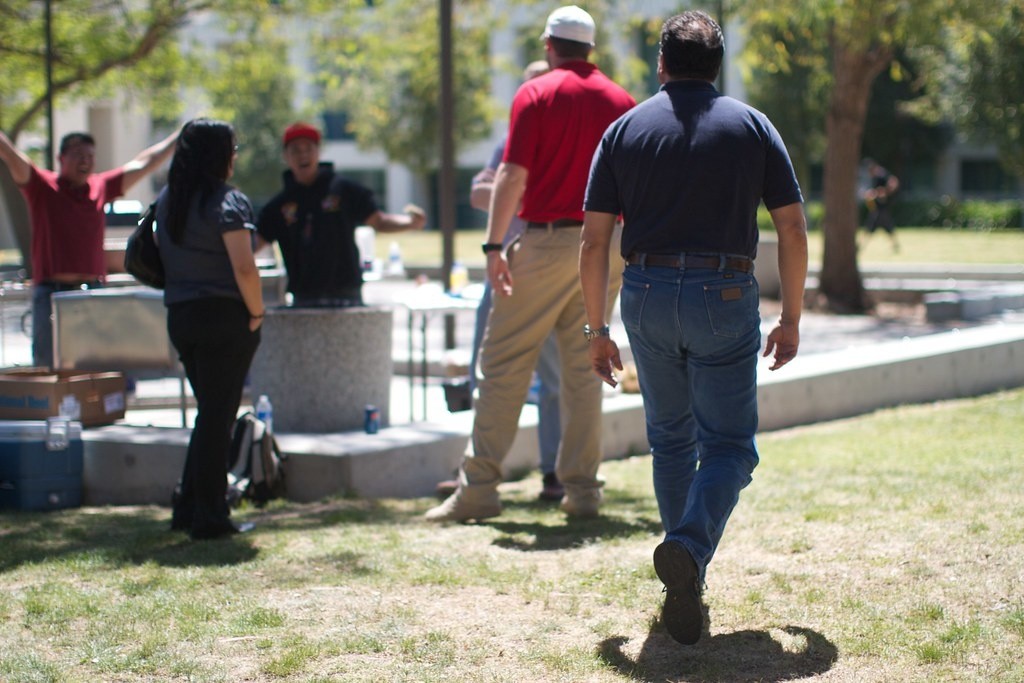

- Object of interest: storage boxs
[442,378,471,411]
[0,372,125,428]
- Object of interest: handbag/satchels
[124,202,166,289]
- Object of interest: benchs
[46,287,251,431]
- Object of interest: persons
[157,118,266,541]
[858,160,901,255]
[0,117,189,371]
[426,0,638,521]
[435,61,569,506]
[254,123,425,307]
[578,0,808,645]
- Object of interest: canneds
[364,405,378,435]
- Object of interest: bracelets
[583,323,610,340]
[480,243,503,252]
[251,313,265,320]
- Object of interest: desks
[404,301,483,422]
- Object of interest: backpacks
[225,406,287,506]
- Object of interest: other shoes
[170,517,193,529]
[653,540,703,645]
[424,486,500,521]
[438,480,458,493]
[192,521,255,540]
[543,473,565,498]
[560,487,602,516]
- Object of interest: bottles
[448,261,470,295]
[389,241,402,274]
[255,394,273,437]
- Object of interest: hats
[284,123,318,146]
[544,5,595,47]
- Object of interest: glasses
[232,144,239,155]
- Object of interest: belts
[527,218,621,229]
[629,253,755,272]
[39,282,90,291]
